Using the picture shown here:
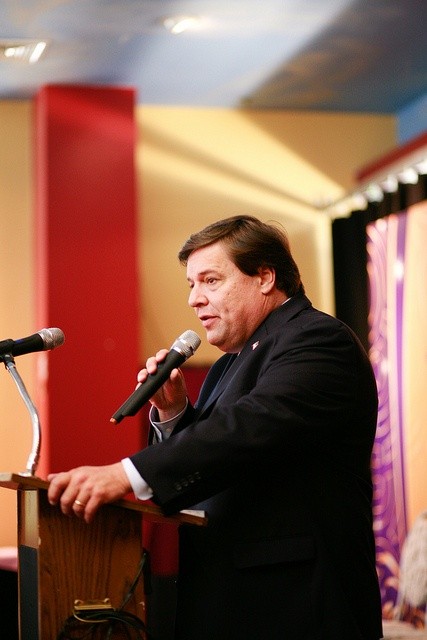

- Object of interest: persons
[48,214,385,640]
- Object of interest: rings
[75,500,86,508]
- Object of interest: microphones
[0,327,65,363]
[109,329,201,426]
